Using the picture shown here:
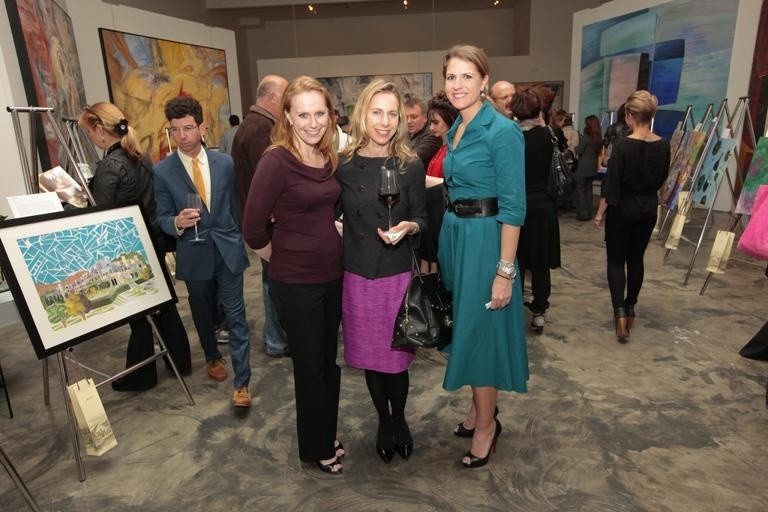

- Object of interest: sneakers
[108,376,156,392]
[216,329,231,344]
[206,353,227,381]
[231,384,252,409]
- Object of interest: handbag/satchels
[64,376,119,458]
[545,149,576,200]
[387,270,454,353]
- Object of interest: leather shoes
[375,415,396,465]
[316,453,343,474]
[390,412,414,461]
[329,437,346,458]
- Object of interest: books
[38,164,88,210]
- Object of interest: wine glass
[186,193,205,243]
[378,167,400,236]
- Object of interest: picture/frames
[0,203,179,359]
[315,71,432,122]
[98,27,231,149]
[512,80,563,111]
[4,0,98,176]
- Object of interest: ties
[190,157,206,205]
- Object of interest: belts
[442,193,506,221]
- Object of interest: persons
[76,100,193,390]
[433,44,535,470]
[334,76,434,466]
[591,90,672,341]
[241,73,346,477]
[231,73,603,360]
[151,93,255,409]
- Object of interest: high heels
[461,415,503,468]
[531,311,544,335]
[614,305,630,340]
[452,404,500,439]
[624,302,635,330]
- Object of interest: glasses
[168,124,200,135]
